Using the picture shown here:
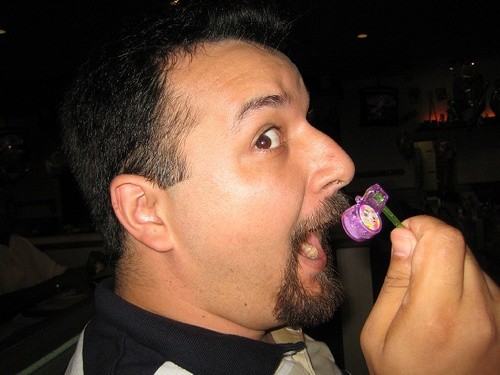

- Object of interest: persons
[50,1,500,375]
